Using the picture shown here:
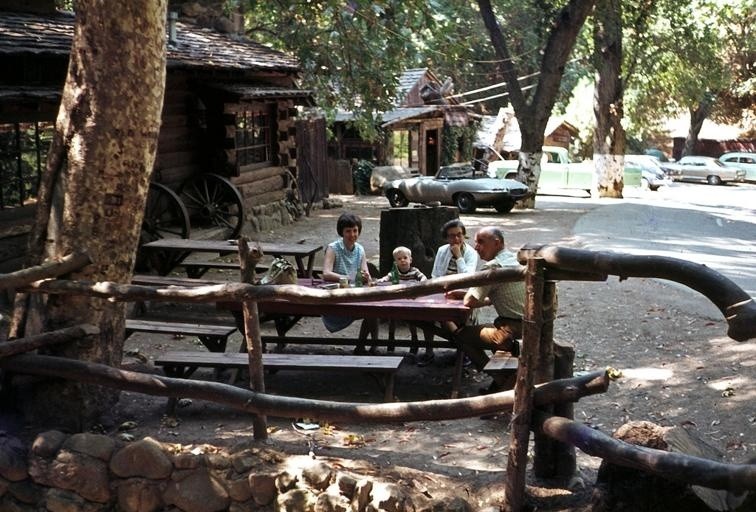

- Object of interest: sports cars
[368,162,533,215]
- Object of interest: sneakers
[463,352,472,366]
[417,353,435,366]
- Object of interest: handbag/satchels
[260,258,299,285]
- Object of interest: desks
[132,239,471,399]
[134,274,471,328]
[140,238,323,264]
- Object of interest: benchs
[178,259,324,281]
[481,349,519,393]
[123,318,403,415]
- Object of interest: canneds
[339,278,348,288]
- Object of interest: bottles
[391,261,400,285]
[355,265,363,287]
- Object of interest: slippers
[369,349,381,355]
[353,348,365,355]
[386,346,395,356]
[408,348,418,360]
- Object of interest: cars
[488,145,756,190]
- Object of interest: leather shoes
[479,378,502,395]
[502,341,519,374]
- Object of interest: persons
[431,216,481,369]
[368,246,427,355]
[319,212,380,357]
[450,225,528,393]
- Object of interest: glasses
[446,233,463,238]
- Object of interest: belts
[499,316,522,323]
